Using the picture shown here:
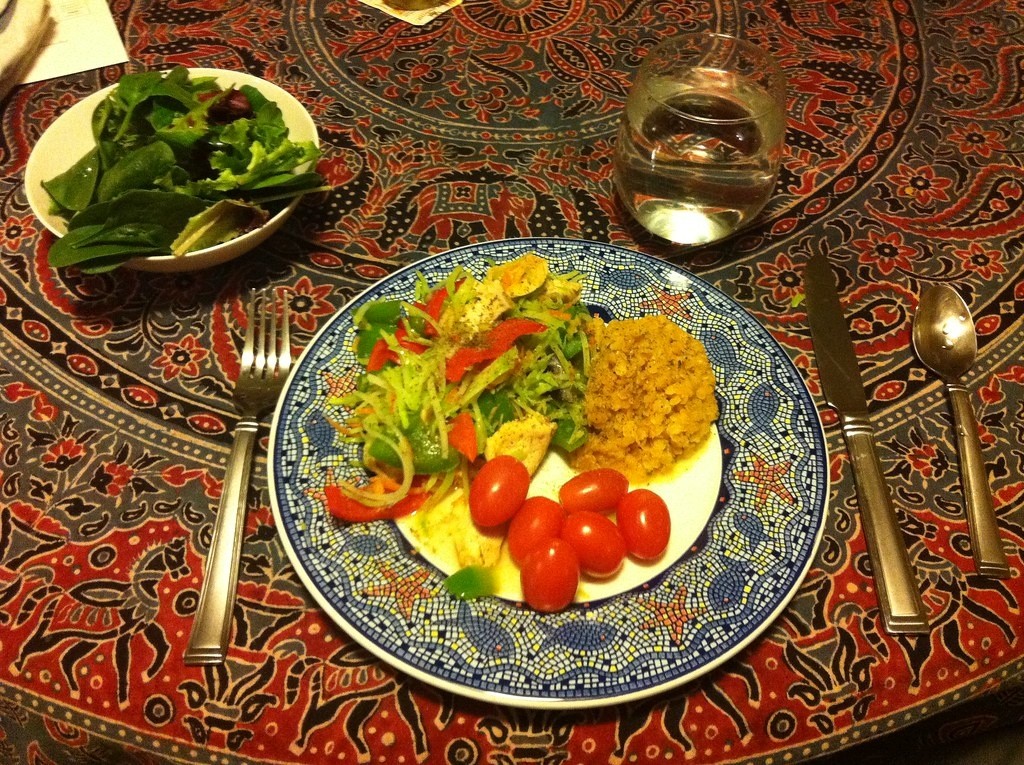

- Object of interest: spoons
[910,284,1012,577]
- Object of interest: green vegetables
[41,67,333,275]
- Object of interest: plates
[265,235,832,713]
[23,65,317,270]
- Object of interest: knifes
[802,253,930,636]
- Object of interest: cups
[614,33,785,247]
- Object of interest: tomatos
[469,456,670,614]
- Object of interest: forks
[183,284,295,667]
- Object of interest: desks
[0,0,1024,765]
